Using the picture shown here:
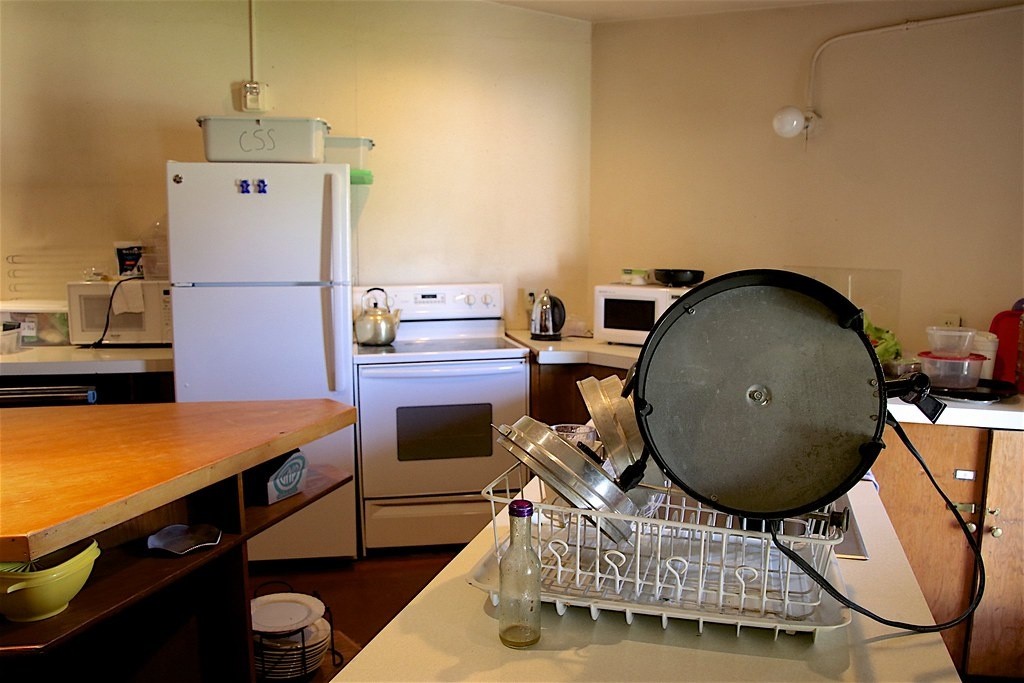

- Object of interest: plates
[148,525,222,555]
[251,593,331,678]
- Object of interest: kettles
[355,287,403,345]
[529,289,566,341]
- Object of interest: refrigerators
[166,160,358,562]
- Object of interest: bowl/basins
[0,538,101,622]
[81,263,105,280]
[885,361,922,380]
[927,326,977,358]
[917,350,987,388]
[620,268,653,286]
[653,268,704,286]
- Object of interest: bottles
[499,500,542,650]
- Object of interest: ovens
[358,365,531,549]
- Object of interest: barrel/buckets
[140,222,168,280]
[971,331,999,379]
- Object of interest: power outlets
[934,314,961,327]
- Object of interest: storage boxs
[195,114,374,169]
[1,300,69,346]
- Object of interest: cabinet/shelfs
[0,398,356,683]
[529,351,630,425]
[871,422,1023,677]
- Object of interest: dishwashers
[0,375,96,408]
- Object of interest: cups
[543,424,596,506]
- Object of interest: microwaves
[67,279,175,345]
[592,282,694,345]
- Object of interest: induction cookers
[352,282,531,364]
